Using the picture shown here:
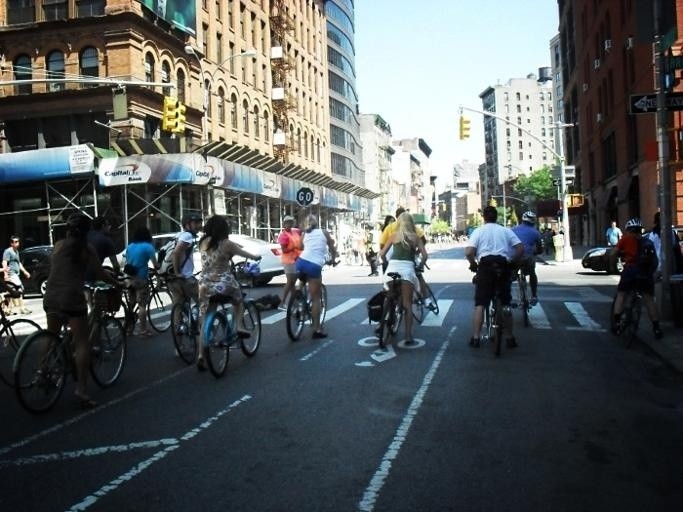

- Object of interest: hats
[283,216,295,221]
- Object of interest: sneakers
[235,328,252,339]
[654,328,663,338]
[614,327,623,335]
[530,296,538,306]
[174,344,191,356]
[469,336,481,348]
[197,354,209,373]
[506,335,519,348]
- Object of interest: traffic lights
[162,95,180,132]
[170,99,187,135]
[457,114,471,142]
[565,193,584,208]
[111,86,132,122]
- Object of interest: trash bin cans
[552,235,564,262]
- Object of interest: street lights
[183,45,262,214]
[502,162,525,173]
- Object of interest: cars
[580,224,682,274]
[100,229,183,274]
[0,244,56,297]
[142,231,287,294]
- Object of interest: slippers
[71,397,98,408]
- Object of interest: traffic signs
[294,186,314,208]
[626,90,682,116]
[661,23,678,52]
[665,55,682,70]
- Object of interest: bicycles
[409,267,441,325]
[374,261,423,351]
[462,263,505,360]
[510,273,535,329]
[603,253,644,350]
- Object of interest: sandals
[122,326,153,337]
[405,338,413,343]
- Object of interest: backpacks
[635,238,659,276]
[367,292,385,323]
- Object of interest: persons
[508,210,544,306]
[195,215,261,372]
[0,266,11,273]
[342,222,378,277]
[293,213,336,338]
[379,207,437,311]
[465,205,524,349]
[438,232,468,247]
[277,215,303,311]
[157,213,203,356]
[381,215,396,233]
[543,228,556,255]
[122,226,159,338]
[86,214,121,361]
[33,212,133,407]
[375,212,428,346]
[2,235,32,315]
[605,211,682,339]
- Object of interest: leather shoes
[312,329,328,338]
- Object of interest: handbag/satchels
[123,264,138,276]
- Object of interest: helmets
[522,211,537,224]
[624,219,644,230]
[182,213,203,225]
